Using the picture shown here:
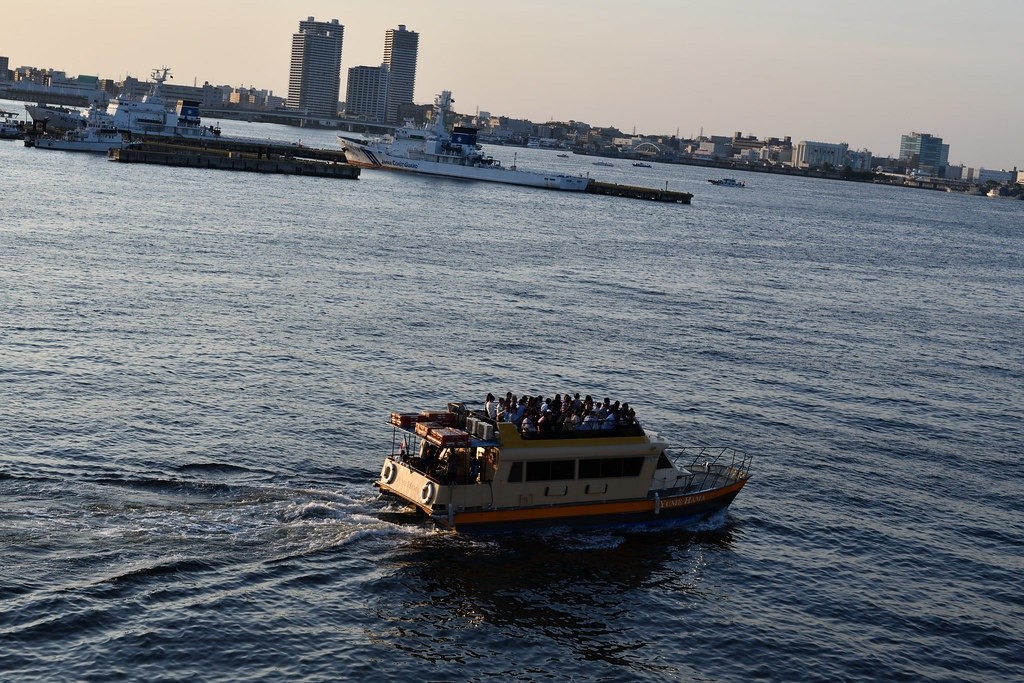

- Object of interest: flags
[398,439,407,451]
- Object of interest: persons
[485,389,637,435]
[398,439,460,483]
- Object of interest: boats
[137,118,164,131]
[591,160,614,167]
[632,162,652,168]
[0,109,24,139]
[23,98,129,153]
[178,116,201,127]
[372,401,755,536]
[337,90,596,191]
[473,157,493,165]
[708,178,745,187]
[441,144,462,153]
[23,65,221,139]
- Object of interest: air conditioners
[476,421,494,441]
[465,416,481,435]
